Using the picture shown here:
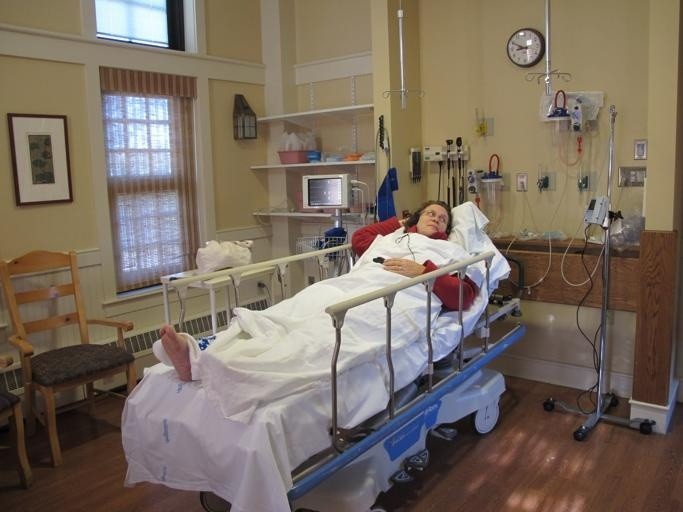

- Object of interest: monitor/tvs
[302,174,351,209]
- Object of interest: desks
[158,260,280,337]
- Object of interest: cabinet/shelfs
[248,103,376,220]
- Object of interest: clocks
[507,27,545,68]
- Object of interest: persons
[157,199,485,383]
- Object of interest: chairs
[0,248,139,489]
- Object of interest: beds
[142,243,525,511]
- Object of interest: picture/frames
[6,112,73,206]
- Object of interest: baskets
[296,237,346,258]
